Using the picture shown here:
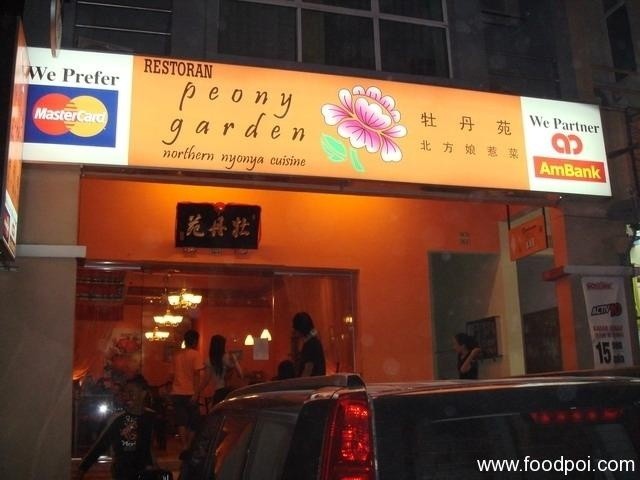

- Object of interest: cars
[176,365,639,478]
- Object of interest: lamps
[144,279,203,342]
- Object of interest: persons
[169,329,203,446]
[291,310,327,376]
[452,333,482,379]
[72,376,172,479]
[198,335,242,405]
